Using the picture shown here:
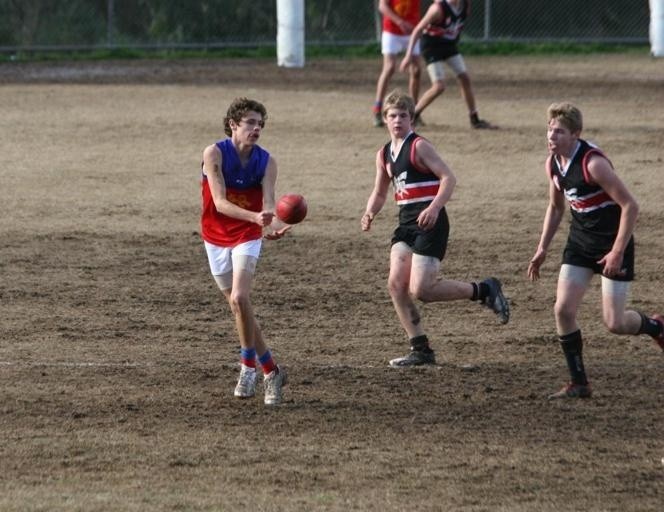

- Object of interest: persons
[357,89,509,367]
[527,104,664,400]
[401,0,499,133]
[200,95,294,407]
[371,1,427,130]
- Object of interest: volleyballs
[275,191,308,225]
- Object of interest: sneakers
[389,344,435,366]
[481,276,510,324]
[652,314,664,350]
[470,119,490,128]
[264,364,288,404]
[233,363,258,399]
[548,383,592,400]
[374,112,383,127]
